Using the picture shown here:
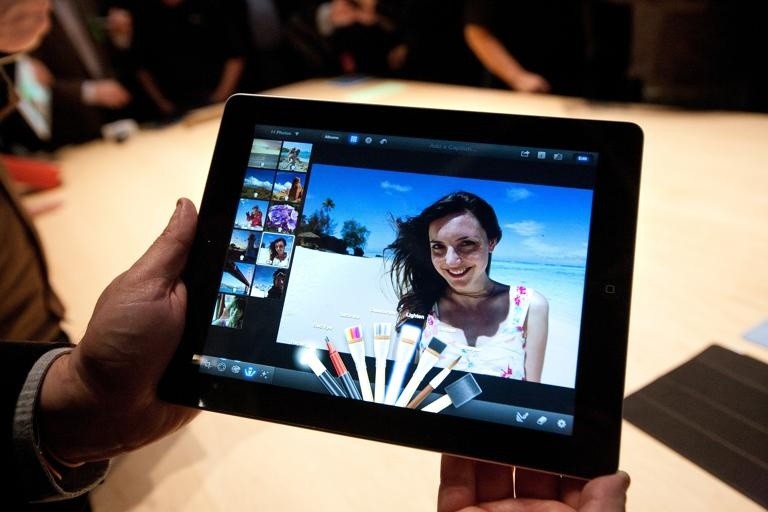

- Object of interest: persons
[211,298,245,329]
[232,286,236,293]
[288,148,302,171]
[239,254,245,263]
[267,221,292,235]
[381,189,548,384]
[0,184,630,511]
[253,191,258,198]
[269,238,290,267]
[284,193,289,201]
[264,269,287,298]
[260,159,265,166]
[245,205,262,228]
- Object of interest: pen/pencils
[30,198,64,220]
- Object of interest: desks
[2,79,768,512]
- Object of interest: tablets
[152,92,644,483]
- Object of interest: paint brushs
[302,323,483,413]
